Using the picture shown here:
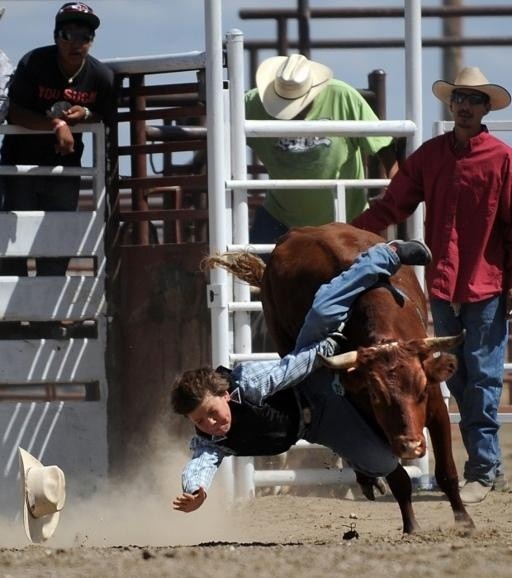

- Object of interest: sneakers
[459,479,493,503]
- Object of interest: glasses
[451,91,484,105]
[59,30,92,45]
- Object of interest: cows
[196,222,476,540]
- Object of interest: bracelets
[83,106,90,122]
[51,119,65,135]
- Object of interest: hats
[56,2,100,30]
[17,446,66,545]
[255,53,333,121]
[431,67,511,111]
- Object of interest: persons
[0,1,114,342]
[349,65,512,506]
[169,238,433,512]
[0,5,14,124]
[245,53,400,264]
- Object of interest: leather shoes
[387,239,433,266]
